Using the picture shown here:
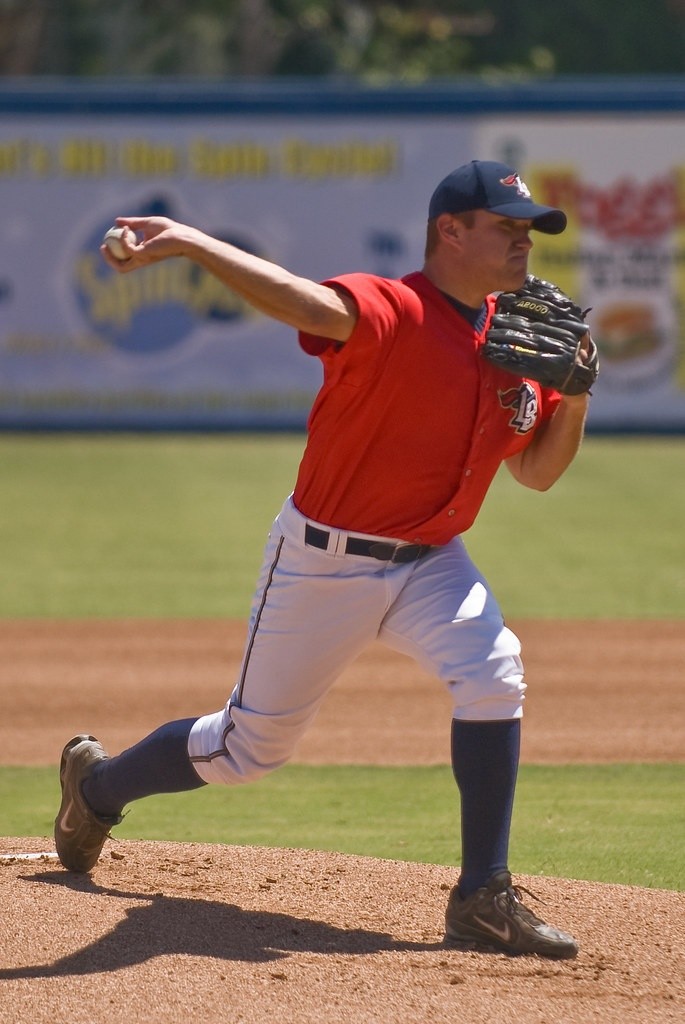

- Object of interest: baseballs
[102,222,147,261]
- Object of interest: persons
[54,160,600,958]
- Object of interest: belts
[306,527,431,565]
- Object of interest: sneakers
[445,871,578,958]
[54,735,132,874]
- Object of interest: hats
[427,160,568,235]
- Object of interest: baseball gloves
[479,275,601,398]
